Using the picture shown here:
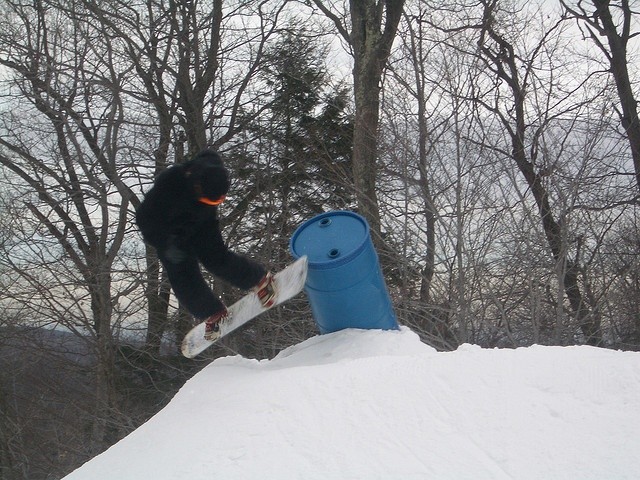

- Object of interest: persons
[134,154,276,336]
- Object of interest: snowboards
[181,254,308,358]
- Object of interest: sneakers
[249,270,279,311]
[204,306,229,341]
